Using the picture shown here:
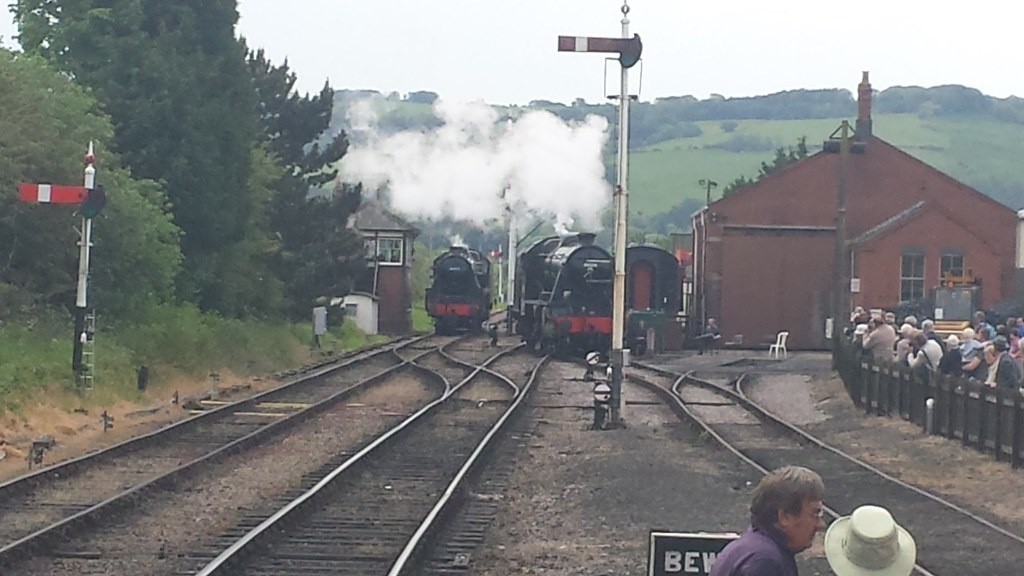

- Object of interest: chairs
[768,331,789,360]
[704,329,721,356]
[724,334,743,357]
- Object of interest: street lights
[698,178,719,204]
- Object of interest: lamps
[594,381,611,402]
[585,351,599,366]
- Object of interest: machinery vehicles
[927,267,984,334]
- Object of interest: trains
[511,234,616,354]
[422,243,494,334]
[624,241,685,317]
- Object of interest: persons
[707,464,825,576]
[847,301,1024,401]
[692,319,722,355]
[820,504,919,576]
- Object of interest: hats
[823,504,916,576]
[853,306,1022,349]
